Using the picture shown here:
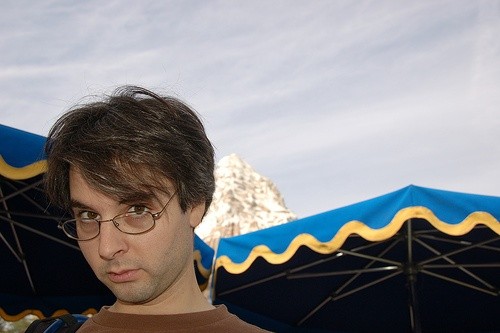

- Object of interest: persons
[44,86,270,333]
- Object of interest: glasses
[57,187,181,241]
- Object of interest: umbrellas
[0,124,215,323]
[211,183,500,333]
